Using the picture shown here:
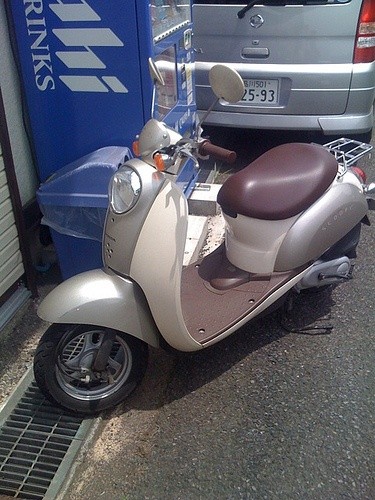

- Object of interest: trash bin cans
[35,145,135,282]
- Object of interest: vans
[190,0,374,151]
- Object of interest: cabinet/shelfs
[11,0,204,199]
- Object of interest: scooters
[34,57,371,413]
